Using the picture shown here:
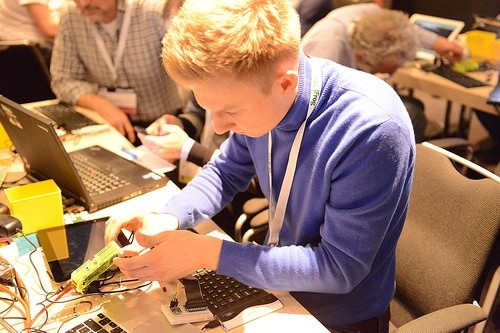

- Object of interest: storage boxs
[2,178,64,238]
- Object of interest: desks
[0,98,332,333]
[387,64,500,152]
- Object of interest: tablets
[36,216,125,284]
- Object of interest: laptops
[0,94,168,214]
[404,13,465,67]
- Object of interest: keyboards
[432,67,489,88]
[180,228,284,330]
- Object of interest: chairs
[0,41,58,104]
[386,142,500,333]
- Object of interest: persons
[0,0,81,105]
[300,2,465,140]
[294,0,333,39]
[102,0,418,333]
[136,0,232,170]
[48,0,192,148]
